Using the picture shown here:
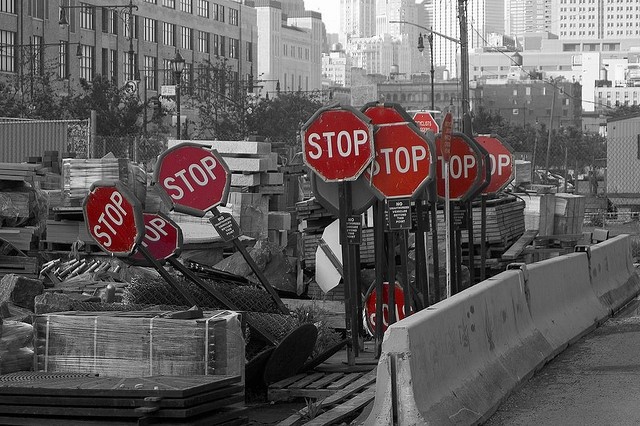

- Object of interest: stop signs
[440,114,452,162]
[412,112,439,134]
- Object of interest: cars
[536,170,576,194]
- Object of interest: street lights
[389,21,471,132]
[170,49,186,139]
[418,27,436,118]
[58,0,135,83]
[20,39,83,117]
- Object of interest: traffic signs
[80,179,145,258]
[458,135,492,202]
[151,140,232,217]
[362,121,435,201]
[359,101,417,132]
[481,132,517,198]
[436,131,482,201]
[300,101,376,184]
[362,276,422,342]
[118,210,184,269]
